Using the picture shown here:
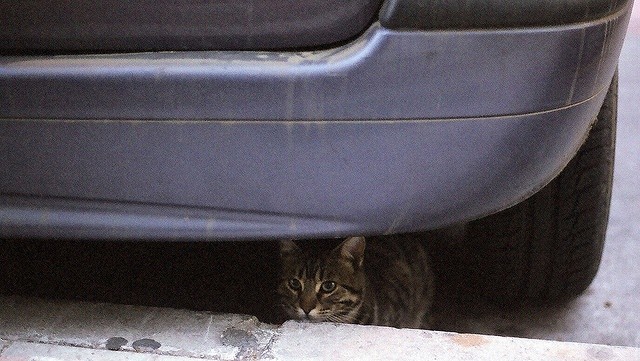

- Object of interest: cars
[1,0,635,306]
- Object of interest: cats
[274,234,436,330]
